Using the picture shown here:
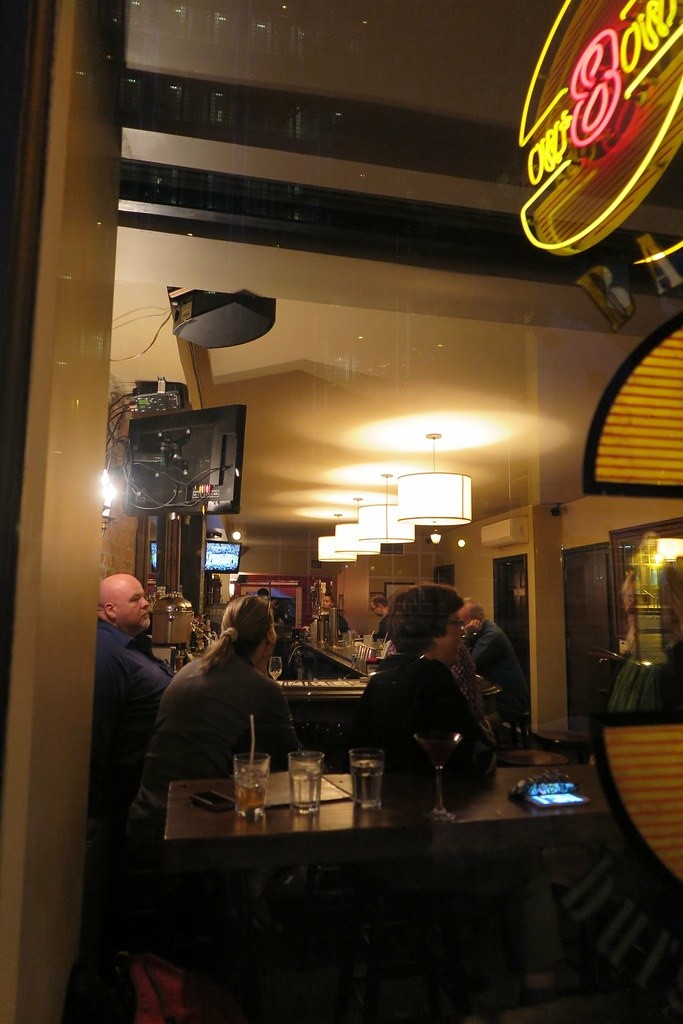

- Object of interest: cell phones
[525,791,590,809]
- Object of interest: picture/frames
[384,582,416,601]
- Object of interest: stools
[497,709,591,767]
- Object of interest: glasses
[448,619,464,631]
[369,606,379,611]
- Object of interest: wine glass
[414,730,463,820]
[269,657,282,681]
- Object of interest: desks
[163,763,622,1024]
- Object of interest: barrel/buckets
[151,596,194,644]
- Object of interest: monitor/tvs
[149,541,156,572]
[124,404,247,516]
[205,539,242,574]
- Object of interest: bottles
[191,613,217,653]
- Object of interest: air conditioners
[480,517,529,549]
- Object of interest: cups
[342,633,386,657]
[288,751,325,814]
[233,753,271,814]
[348,748,384,808]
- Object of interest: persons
[92,574,393,917]
[457,599,528,750]
[353,584,572,1003]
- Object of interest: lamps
[335,498,380,555]
[318,514,357,562]
[359,474,415,544]
[397,433,472,525]
